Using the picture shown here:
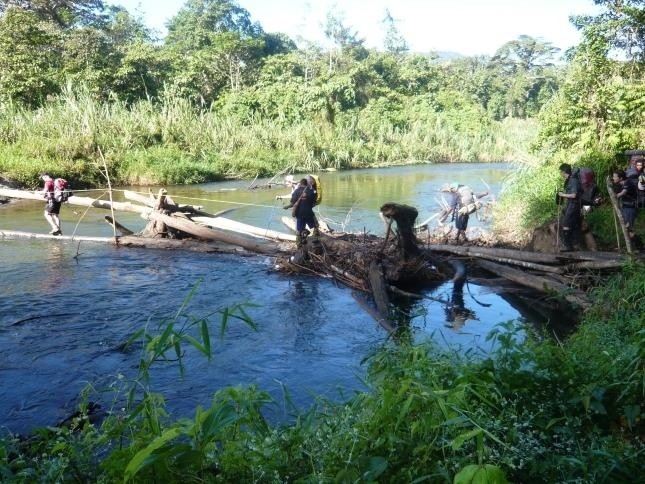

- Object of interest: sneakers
[560,245,573,252]
[49,229,62,236]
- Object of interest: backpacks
[580,168,596,203]
[54,178,68,202]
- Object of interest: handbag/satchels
[292,198,300,217]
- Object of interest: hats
[39,173,49,180]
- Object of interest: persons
[439,183,482,245]
[283,179,320,247]
[164,195,178,212]
[380,202,422,262]
[38,172,62,236]
[275,180,320,244]
[556,149,644,252]
